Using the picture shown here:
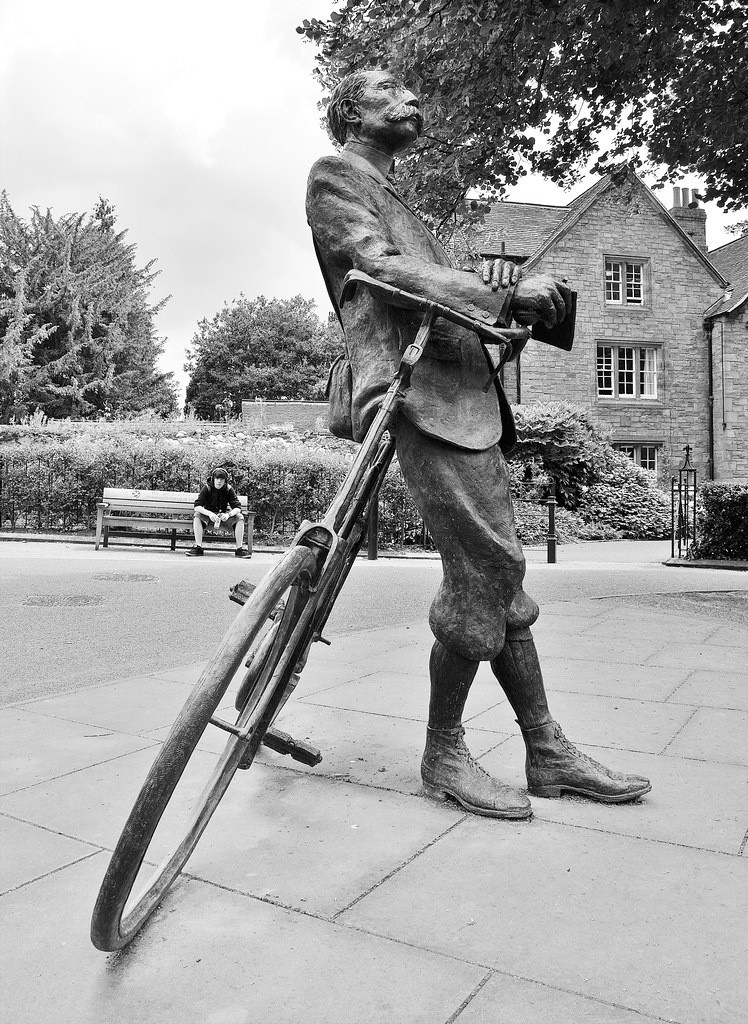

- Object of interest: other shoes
[235,547,251,558]
[185,545,204,556]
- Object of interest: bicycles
[87,265,536,957]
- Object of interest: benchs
[94,487,257,556]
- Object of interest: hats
[212,468,228,483]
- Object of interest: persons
[308,68,656,817]
[185,468,252,559]
